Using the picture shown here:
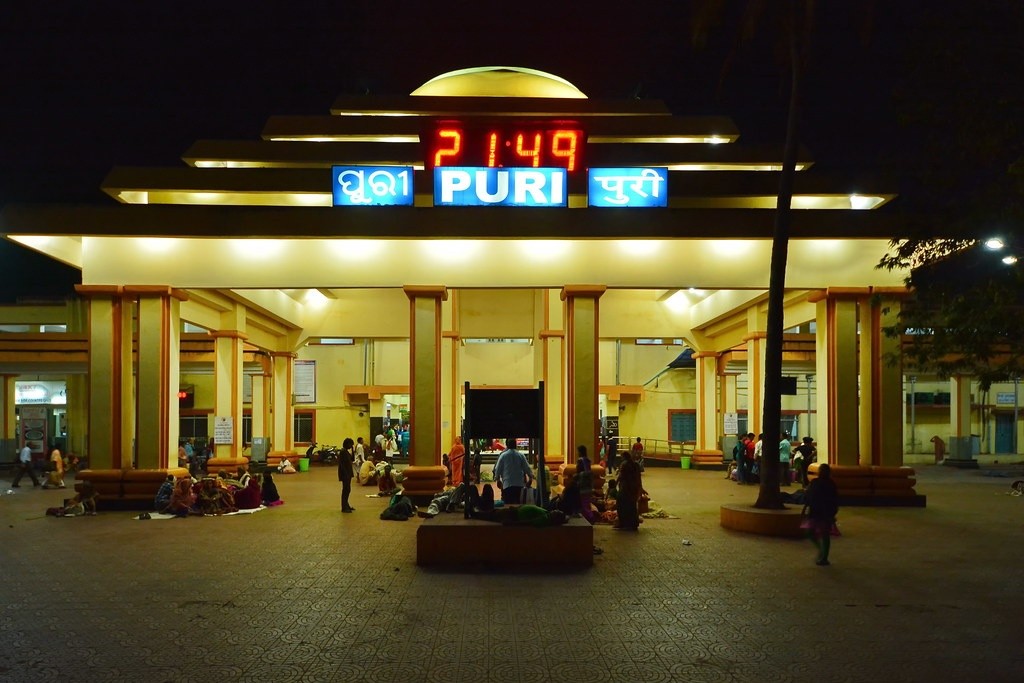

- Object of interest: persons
[154,438,280,517]
[725,430,817,488]
[801,464,839,566]
[11,441,78,489]
[337,421,644,532]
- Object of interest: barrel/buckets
[300,459,309,471]
[681,457,691,469]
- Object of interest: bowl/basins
[25,421,43,439]
